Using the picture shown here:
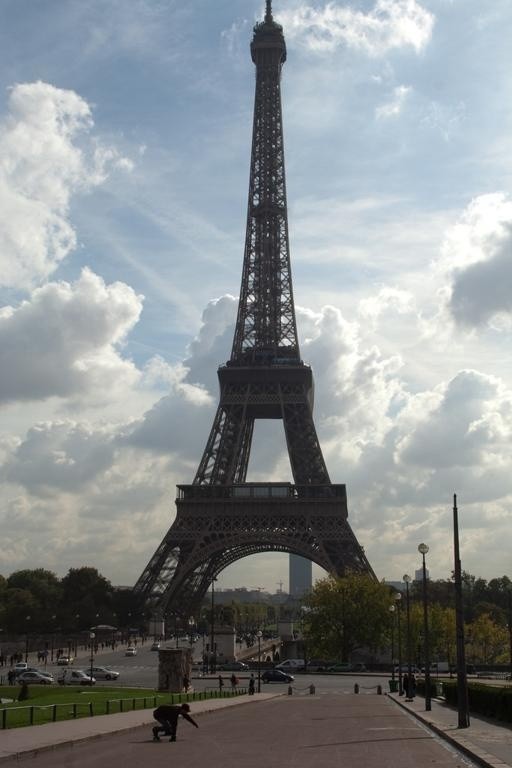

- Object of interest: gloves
[169,736,176,742]
[194,723,198,728]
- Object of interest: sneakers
[153,727,158,736]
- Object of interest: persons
[8,669,16,686]
[152,700,199,743]
[230,673,237,692]
[0,629,279,665]
[248,673,255,696]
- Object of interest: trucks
[274,659,306,671]
[421,662,449,673]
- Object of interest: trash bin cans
[388,680,400,693]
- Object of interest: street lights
[21,612,197,661]
[416,542,433,713]
[255,630,262,693]
[389,605,395,681]
[394,592,403,696]
[402,574,413,699]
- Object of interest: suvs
[261,670,296,684]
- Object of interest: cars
[12,656,121,687]
[328,663,371,674]
[394,664,418,674]
[297,661,326,672]
[218,661,249,672]
[236,635,243,643]
[182,634,199,644]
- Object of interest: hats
[182,704,191,712]
